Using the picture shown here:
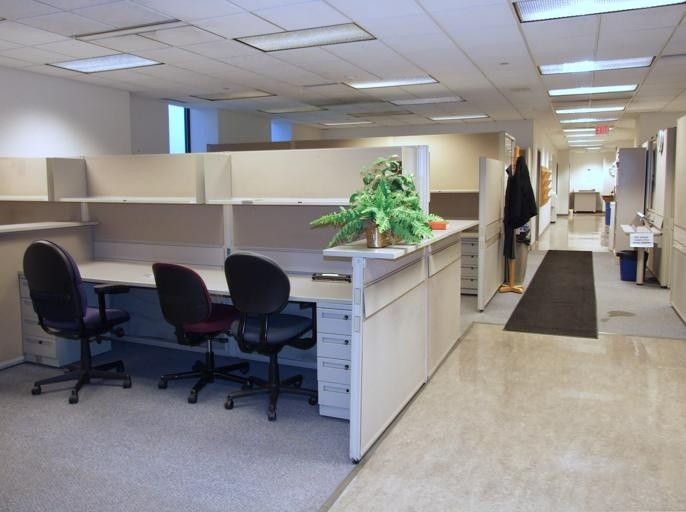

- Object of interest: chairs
[149,261,254,403]
[222,250,316,420]
[21,240,133,401]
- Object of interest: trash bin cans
[505,229,531,284]
[617,250,647,282]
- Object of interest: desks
[17,145,425,462]
[621,223,662,284]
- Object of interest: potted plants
[422,211,448,230]
[307,151,432,248]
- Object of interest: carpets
[501,249,597,338]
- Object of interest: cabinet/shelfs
[460,232,479,294]
[611,146,648,250]
